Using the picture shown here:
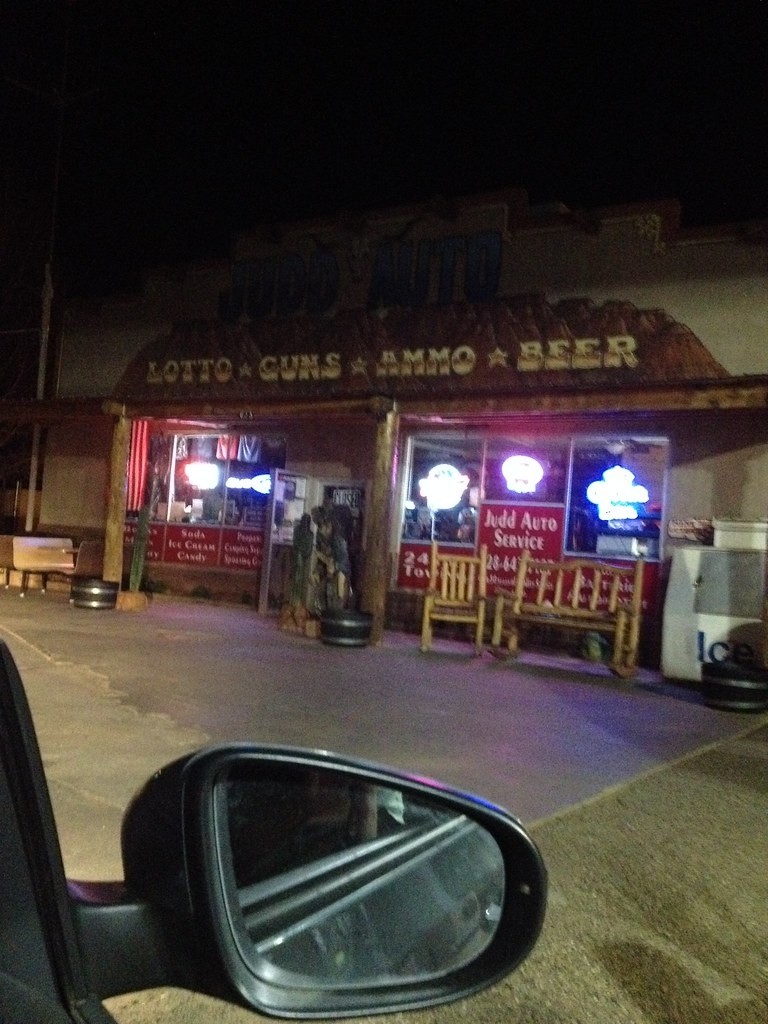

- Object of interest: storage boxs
[712,519,768,549]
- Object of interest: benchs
[56,541,152,604]
[0,535,74,597]
[493,552,644,669]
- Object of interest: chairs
[421,541,488,657]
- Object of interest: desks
[65,549,80,595]
